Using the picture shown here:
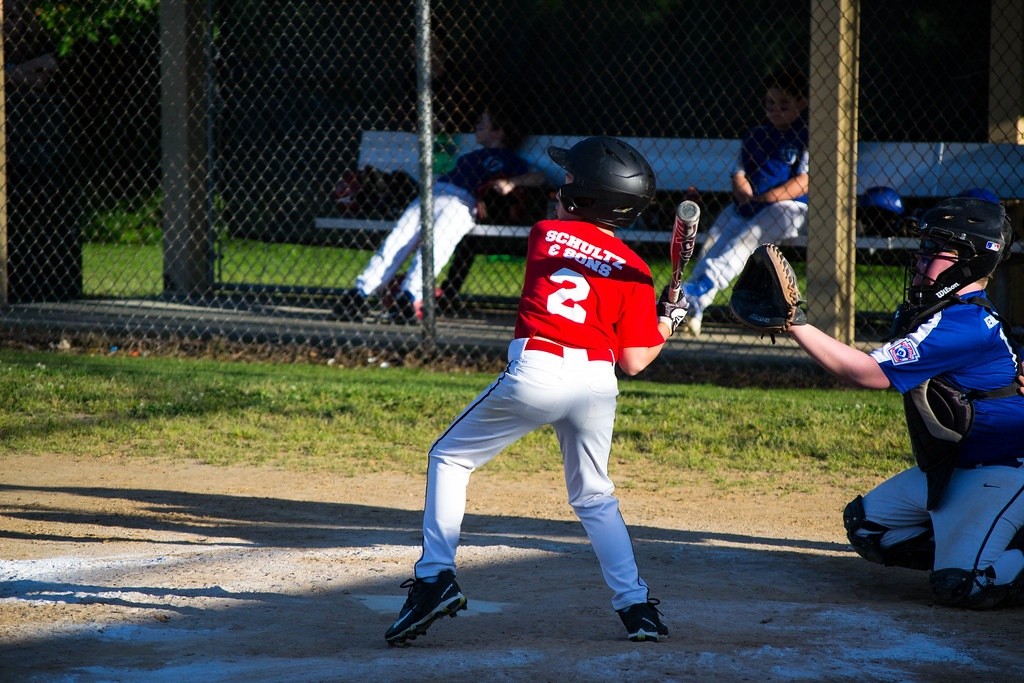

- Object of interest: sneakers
[385,571,467,645]
[620,597,669,642]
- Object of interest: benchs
[315,129,1023,291]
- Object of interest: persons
[384,138,690,646]
[784,198,1024,611]
[327,101,546,325]
[675,70,809,337]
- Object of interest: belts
[525,340,613,362]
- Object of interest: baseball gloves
[729,242,809,345]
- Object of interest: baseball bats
[668,199,701,304]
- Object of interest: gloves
[657,284,688,332]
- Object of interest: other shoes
[332,289,369,318]
[680,305,704,338]
[368,292,415,325]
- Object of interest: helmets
[905,199,1013,304]
[549,136,657,229]
